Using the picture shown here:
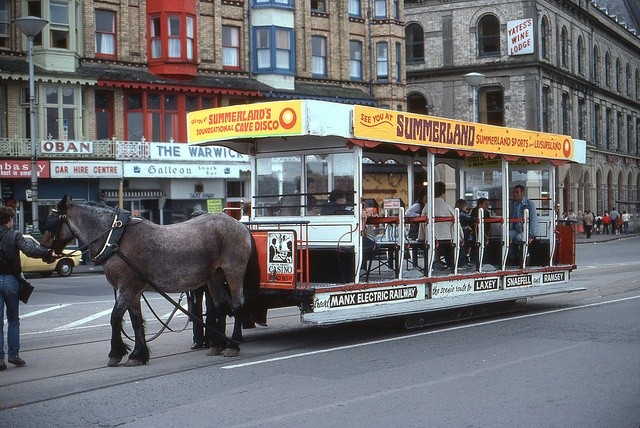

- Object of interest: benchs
[436,217,455,274]
[526,240,549,265]
[297,244,355,256]
[460,218,480,273]
[365,217,398,280]
[510,218,525,268]
[404,216,429,277]
[484,218,504,270]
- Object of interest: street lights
[10,16,49,243]
[460,72,487,123]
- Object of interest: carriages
[39,98,587,366]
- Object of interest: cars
[18,234,83,277]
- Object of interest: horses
[39,193,260,367]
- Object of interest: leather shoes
[204,343,211,348]
[190,342,203,349]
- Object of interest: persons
[405,186,427,237]
[187,208,218,351]
[319,189,352,215]
[417,182,466,269]
[466,197,491,264]
[1,205,55,369]
[453,198,475,266]
[261,175,318,216]
[560,207,631,238]
[509,184,539,264]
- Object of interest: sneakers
[0,356,7,369]
[8,354,25,365]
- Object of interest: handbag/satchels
[16,274,33,303]
